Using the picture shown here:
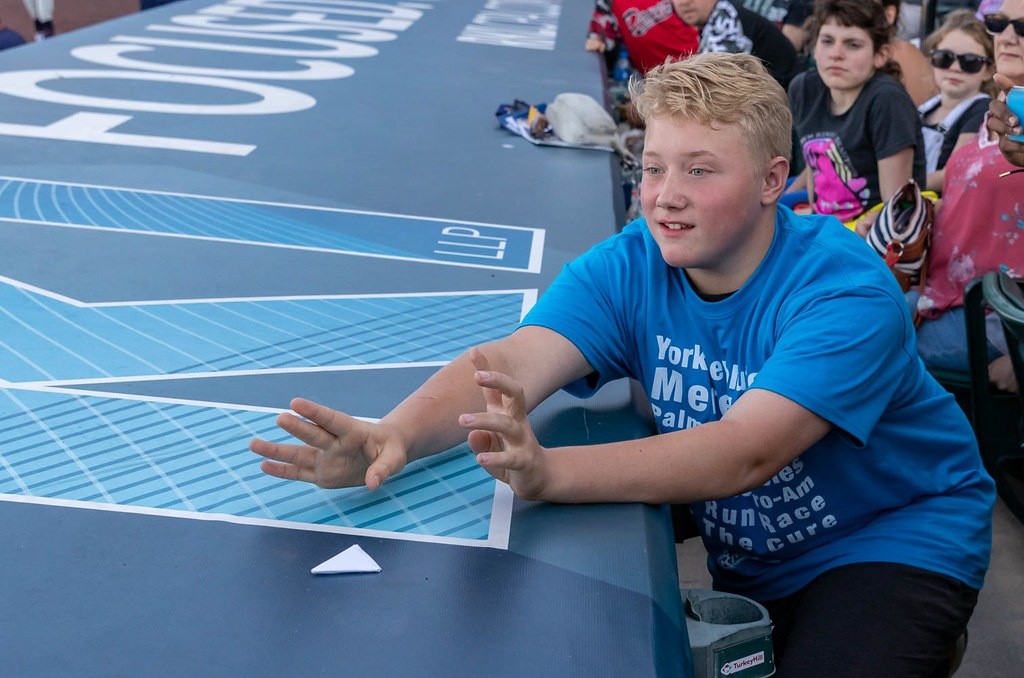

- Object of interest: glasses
[984,13,1024,38]
[929,49,993,74]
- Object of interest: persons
[583,0,1024,394]
[249,53,997,678]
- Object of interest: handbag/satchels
[865,178,934,292]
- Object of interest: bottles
[615,51,631,83]
